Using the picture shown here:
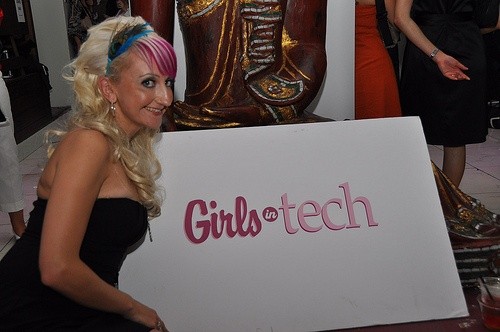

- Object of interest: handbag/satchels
[375,0,401,49]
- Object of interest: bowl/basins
[478,277,500,308]
[476,294,500,332]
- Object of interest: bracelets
[430,48,439,58]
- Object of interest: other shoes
[14,233,21,239]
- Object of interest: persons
[355,0,402,120]
[131,0,500,242]
[0,9,177,332]
[0,70,27,240]
[394,0,489,188]
[68,0,129,49]
